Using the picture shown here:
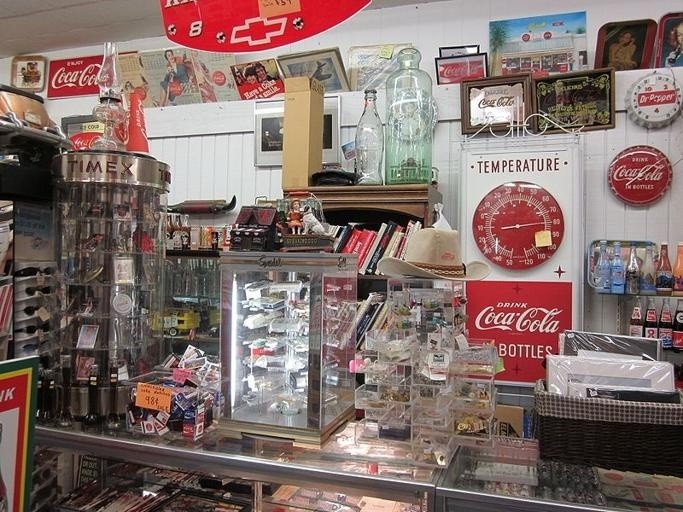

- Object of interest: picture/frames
[347,43,413,92]
[277,46,348,91]
[529,69,615,133]
[253,94,339,167]
[593,19,656,71]
[435,52,488,85]
[9,53,46,92]
[653,11,683,68]
[460,73,535,135]
[438,45,481,57]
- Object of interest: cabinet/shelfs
[279,182,441,384]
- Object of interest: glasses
[14,323,52,336]
[13,306,44,315]
[17,340,51,356]
[15,286,52,297]
[16,266,53,277]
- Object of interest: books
[321,218,424,276]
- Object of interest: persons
[152,50,199,108]
[233,63,274,85]
[281,188,336,251]
[607,30,638,71]
[663,22,683,66]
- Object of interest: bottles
[671,299,682,353]
[673,242,682,297]
[354,89,383,185]
[172,214,182,249]
[609,241,624,292]
[165,258,219,298]
[629,295,643,339]
[658,298,672,351]
[640,241,656,296]
[199,224,234,249]
[624,241,638,295]
[594,239,610,292]
[166,214,174,248]
[643,298,658,339]
[656,242,673,295]
[180,215,190,249]
[384,48,432,185]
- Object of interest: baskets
[534,380,683,479]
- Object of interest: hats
[290,198,299,206]
[378,228,490,282]
